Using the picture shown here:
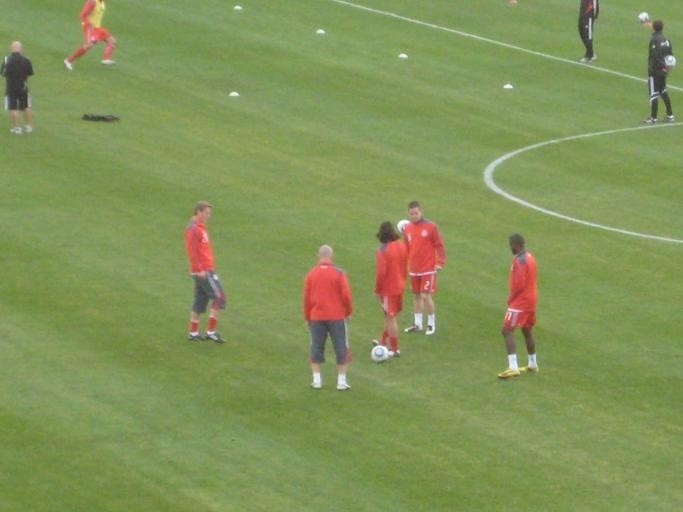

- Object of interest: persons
[577,0,598,62]
[303,245,351,391]
[0,40,34,134]
[496,233,539,379]
[184,202,226,344]
[642,20,675,123]
[402,201,445,336]
[63,0,116,70]
[371,221,408,357]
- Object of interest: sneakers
[187,331,202,342]
[498,368,520,378]
[336,382,351,391]
[519,366,541,373]
[371,340,386,347]
[664,115,676,122]
[22,125,32,133]
[203,331,222,343]
[63,58,74,70]
[387,349,400,356]
[10,127,22,134]
[99,59,117,64]
[643,114,659,125]
[580,54,596,63]
[425,325,436,336]
[310,381,322,389]
[404,324,422,333]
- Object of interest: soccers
[664,55,676,66]
[370,346,389,362]
[395,220,408,234]
[639,11,649,22]
